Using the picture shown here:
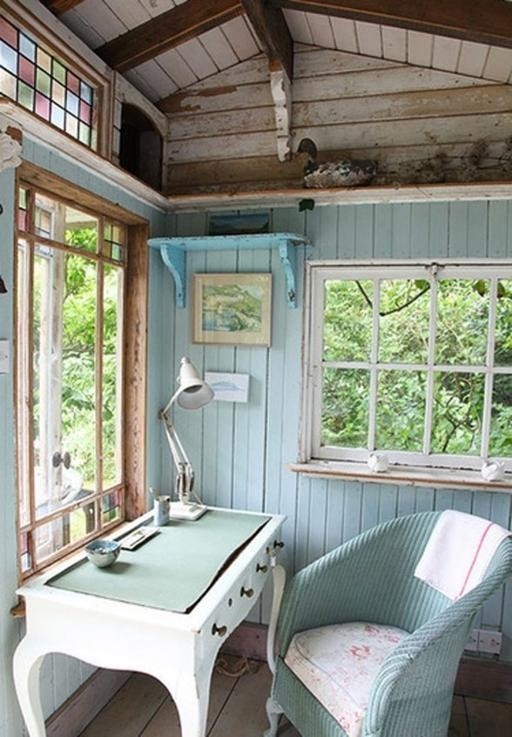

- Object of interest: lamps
[157,353,213,522]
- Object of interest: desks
[10,502,288,737]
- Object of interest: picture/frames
[194,271,275,347]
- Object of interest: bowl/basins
[85,539,120,567]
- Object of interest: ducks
[294,137,379,188]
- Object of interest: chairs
[264,510,512,737]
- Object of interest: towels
[413,510,512,600]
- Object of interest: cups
[153,495,170,527]
